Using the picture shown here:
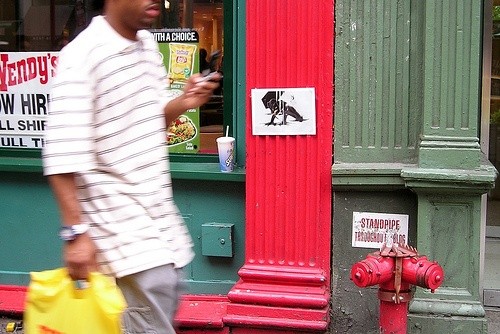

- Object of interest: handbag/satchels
[24,268,125,334]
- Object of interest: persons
[199,48,209,72]
[42,0,222,334]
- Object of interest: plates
[167,115,198,147]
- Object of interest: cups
[216,136,236,174]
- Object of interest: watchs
[58,223,89,242]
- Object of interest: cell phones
[198,49,221,82]
